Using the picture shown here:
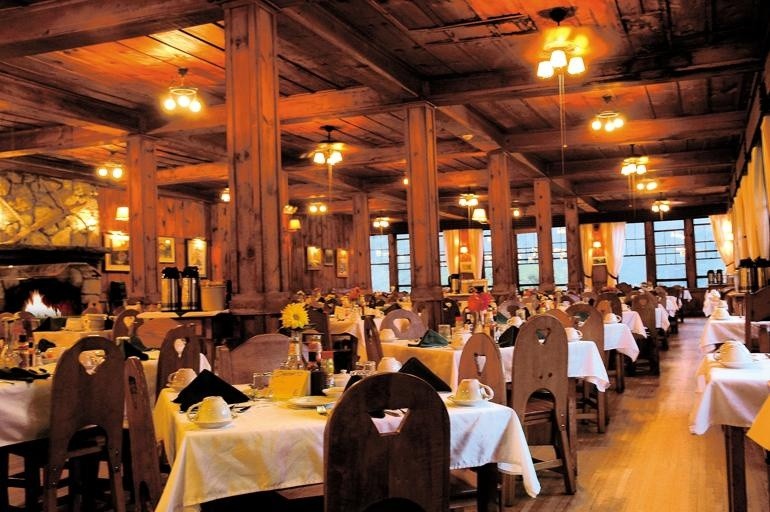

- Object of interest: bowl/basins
[322,386,345,397]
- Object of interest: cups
[564,295,753,367]
[187,394,232,422]
[454,378,494,401]
[166,368,198,384]
[377,356,402,372]
[438,324,450,340]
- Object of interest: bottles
[452,303,546,348]
[16,335,41,368]
[359,295,367,315]
[253,335,335,390]
[343,296,351,318]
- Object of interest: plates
[289,396,337,407]
[194,421,232,429]
[166,383,186,392]
[447,394,493,406]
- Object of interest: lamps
[219,180,230,203]
[313,125,345,165]
[621,144,648,175]
[537,7,585,78]
[373,210,389,228]
[471,208,489,224]
[96,150,124,179]
[637,174,657,190]
[116,207,129,221]
[163,68,201,112]
[592,94,623,132]
[459,186,478,206]
[652,192,669,212]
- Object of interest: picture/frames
[157,234,176,264]
[103,232,130,274]
[305,245,351,278]
[184,237,209,279]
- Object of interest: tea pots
[755,256,770,291]
[716,270,722,283]
[708,270,714,284]
[736,257,755,292]
